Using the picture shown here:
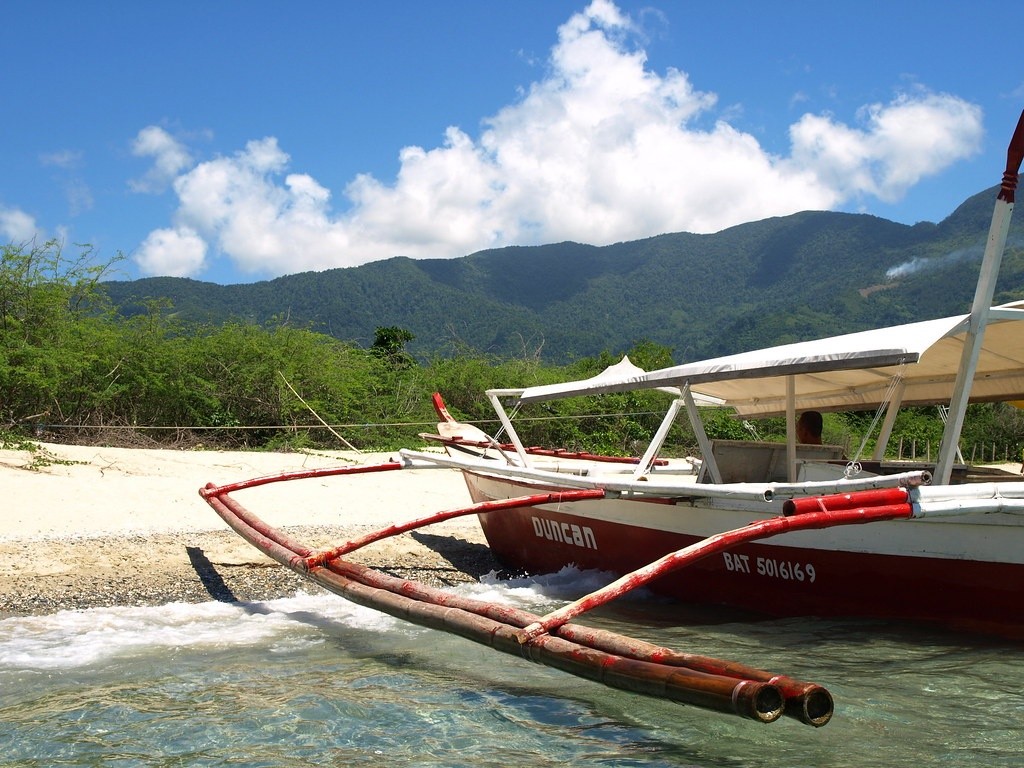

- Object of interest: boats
[201,298,1024,728]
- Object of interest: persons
[796,411,823,444]
